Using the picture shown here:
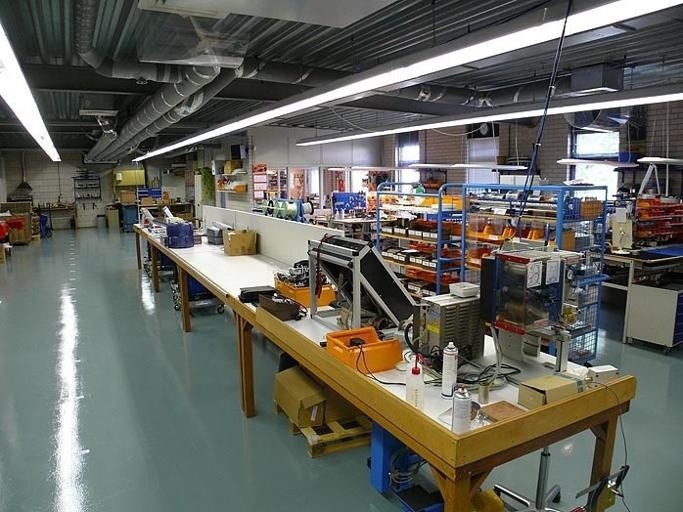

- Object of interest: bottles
[440,341,458,400]
[404,358,426,411]
[450,385,472,435]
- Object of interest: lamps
[131,0,682,162]
[294,57,683,147]
[0,23,62,162]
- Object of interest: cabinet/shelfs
[73,174,101,201]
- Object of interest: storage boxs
[121,190,135,204]
[140,198,153,205]
